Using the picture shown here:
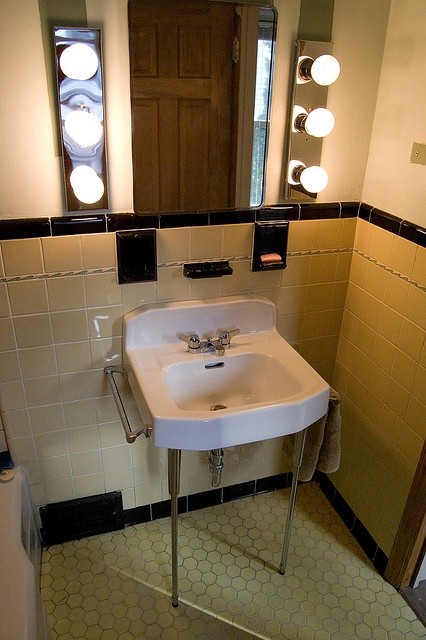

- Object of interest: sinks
[122,295,330,453]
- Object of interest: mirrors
[126,0,278,216]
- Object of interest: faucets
[208,341,225,356]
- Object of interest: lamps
[60,41,99,82]
[295,108,335,137]
[70,166,104,205]
[300,55,341,87]
[63,110,103,149]
[292,165,328,193]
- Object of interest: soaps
[260,253,282,261]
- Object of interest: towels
[283,387,342,482]
